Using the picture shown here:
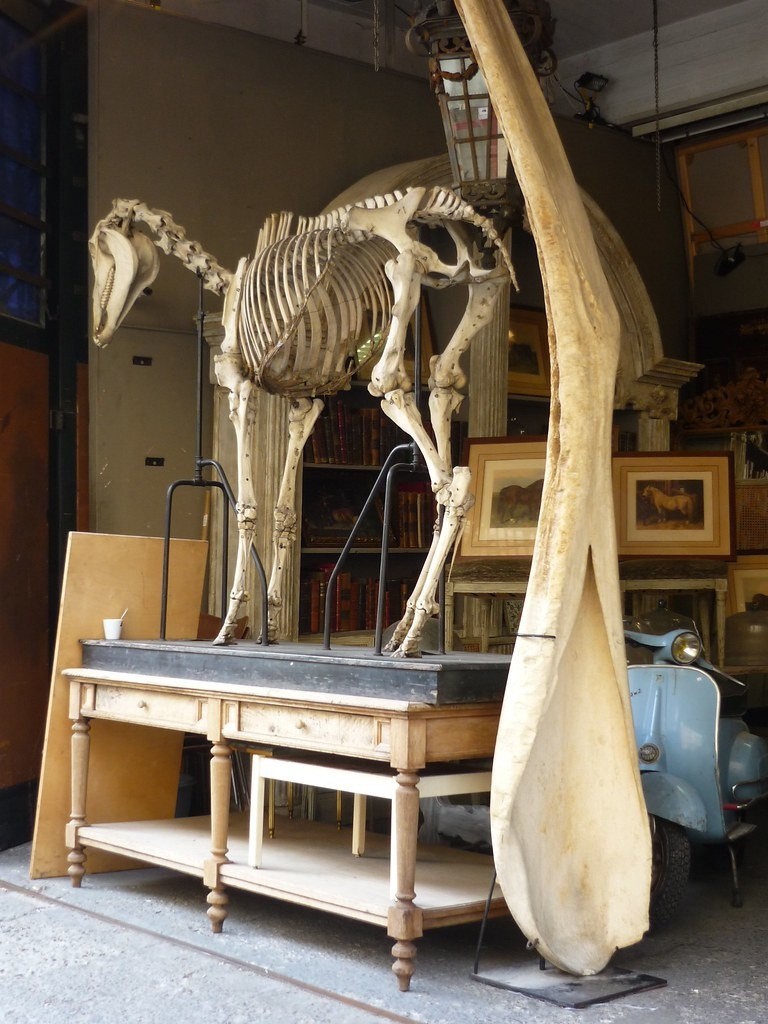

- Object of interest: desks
[62,667,501,993]
[247,749,491,868]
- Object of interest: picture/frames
[724,554,768,618]
[465,434,548,559]
[611,451,736,560]
[356,291,440,385]
[508,309,550,395]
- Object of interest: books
[301,481,441,548]
[304,395,467,470]
[293,567,416,635]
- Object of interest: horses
[641,486,693,524]
[497,478,544,525]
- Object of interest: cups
[103,619,123,640]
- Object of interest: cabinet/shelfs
[290,380,470,645]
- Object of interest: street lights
[405,1,547,650]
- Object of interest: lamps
[407,0,557,270]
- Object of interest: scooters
[623,627,768,937]
